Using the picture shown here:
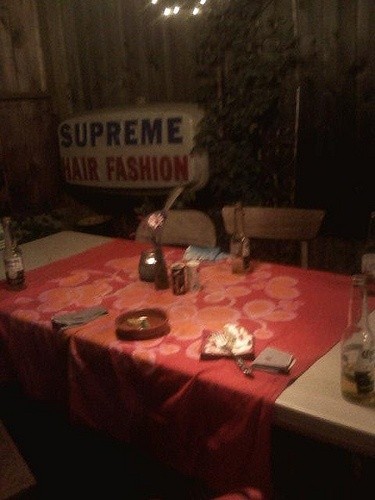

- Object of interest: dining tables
[1,231,375,457]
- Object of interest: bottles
[230,203,250,275]
[1,217,25,291]
[339,277,374,403]
[138,250,163,280]
[153,245,168,290]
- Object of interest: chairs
[223,206,325,272]
[139,210,219,250]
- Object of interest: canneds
[170,261,201,296]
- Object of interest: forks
[213,330,252,375]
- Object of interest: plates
[198,328,254,360]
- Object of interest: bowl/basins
[115,308,169,340]
